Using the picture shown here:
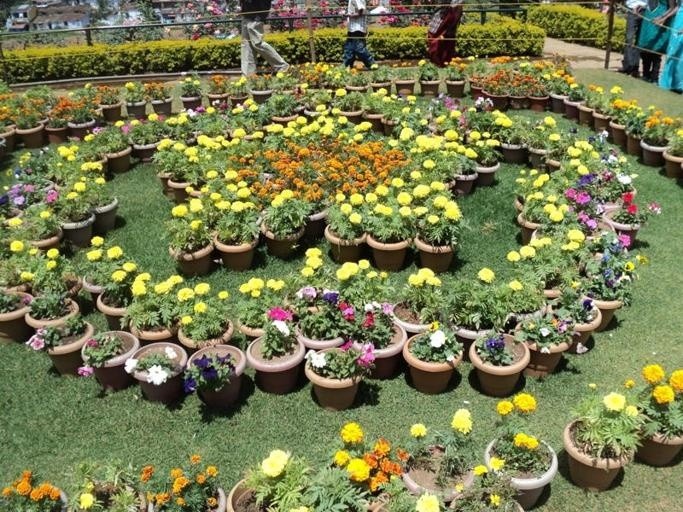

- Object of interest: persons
[341,0,379,72]
[652,0,682,96]
[237,0,288,77]
[616,1,648,78]
[630,0,675,82]
[424,1,466,69]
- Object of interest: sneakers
[616,67,659,84]
